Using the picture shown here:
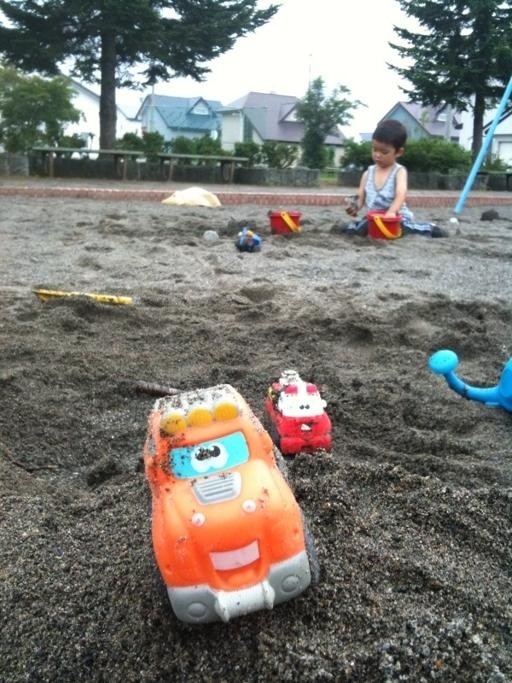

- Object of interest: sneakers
[342,220,360,232]
[430,223,448,238]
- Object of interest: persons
[342,119,448,238]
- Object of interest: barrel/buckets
[366,209,403,241]
[268,208,301,233]
